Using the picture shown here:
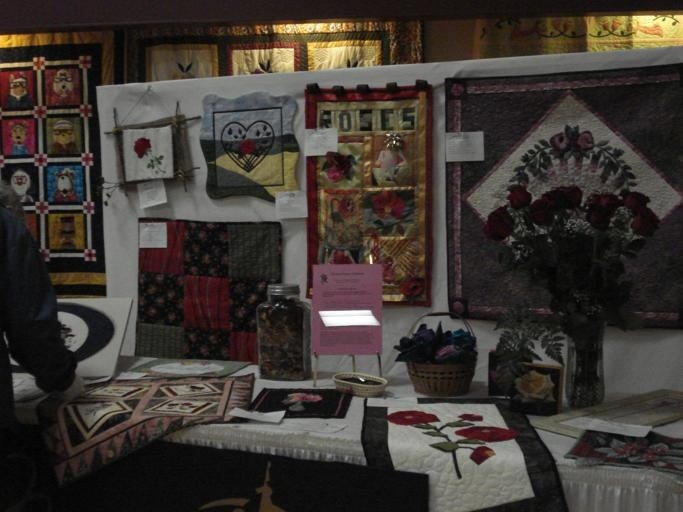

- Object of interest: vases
[550,309,612,409]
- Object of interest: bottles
[255,283,313,383]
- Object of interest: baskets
[406,312,478,395]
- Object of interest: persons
[0,206,83,508]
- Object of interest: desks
[9,353,681,511]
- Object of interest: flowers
[481,178,660,321]
[392,323,479,380]
